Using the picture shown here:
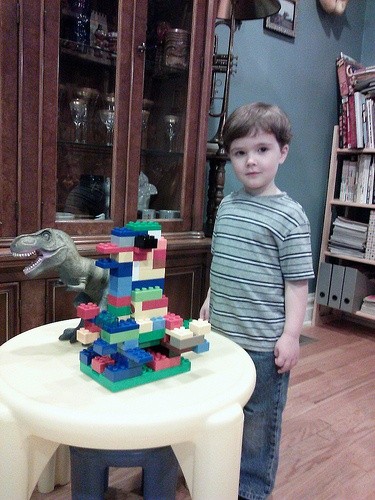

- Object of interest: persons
[198,101,315,500]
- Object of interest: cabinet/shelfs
[0,0,218,345]
[311,125,375,326]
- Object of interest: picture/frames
[264,0,296,40]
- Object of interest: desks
[0,318,257,500]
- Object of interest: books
[360,294,375,315]
[338,153,375,204]
[336,51,375,149]
[327,209,375,260]
